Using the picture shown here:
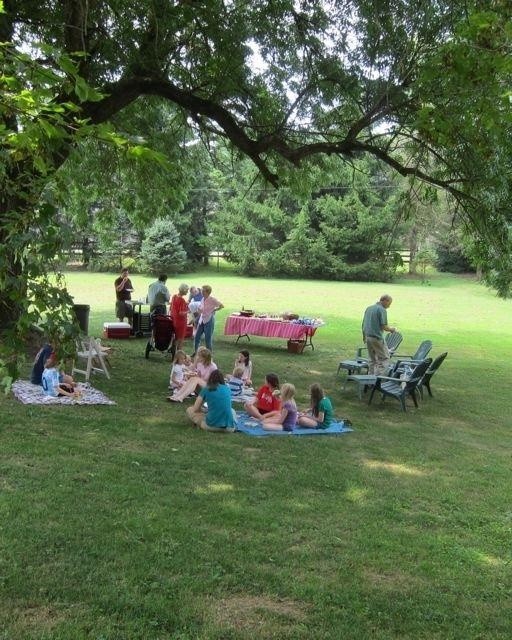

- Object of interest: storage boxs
[287,339,306,353]
[185,320,193,338]
[103,322,132,339]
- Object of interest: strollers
[144,305,177,362]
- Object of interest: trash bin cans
[72,304,90,337]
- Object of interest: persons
[148,273,171,314]
[259,383,298,431]
[31,341,79,399]
[361,295,397,390]
[114,269,136,326]
[226,349,253,386]
[186,369,237,433]
[165,283,225,403]
[228,367,253,396]
[244,373,281,422]
[297,383,335,429]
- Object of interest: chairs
[336,329,447,413]
[71,335,113,381]
